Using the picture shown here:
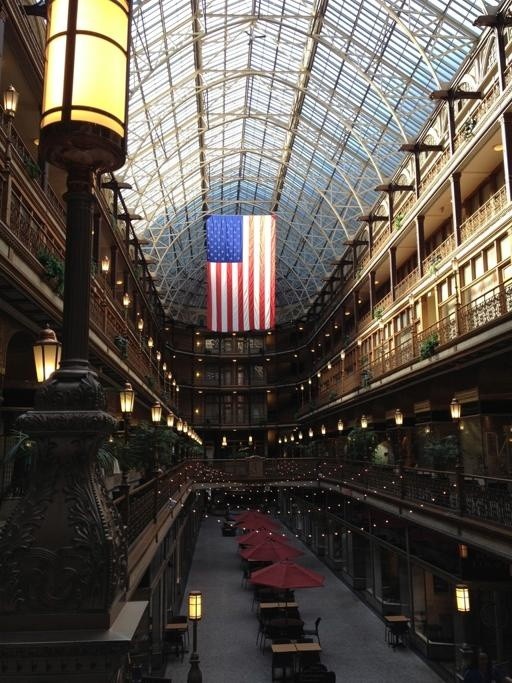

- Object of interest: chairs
[382,610,410,649]
[256,598,336,682]
[163,615,190,663]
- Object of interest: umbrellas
[232,510,326,623]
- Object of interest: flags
[205,213,278,333]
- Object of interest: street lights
[187,591,203,683]
[454,583,478,683]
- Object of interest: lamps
[29,320,65,386]
[95,250,134,328]
[34,1,134,176]
[117,382,134,424]
[151,400,204,446]
[277,397,463,445]
[1,84,20,122]
